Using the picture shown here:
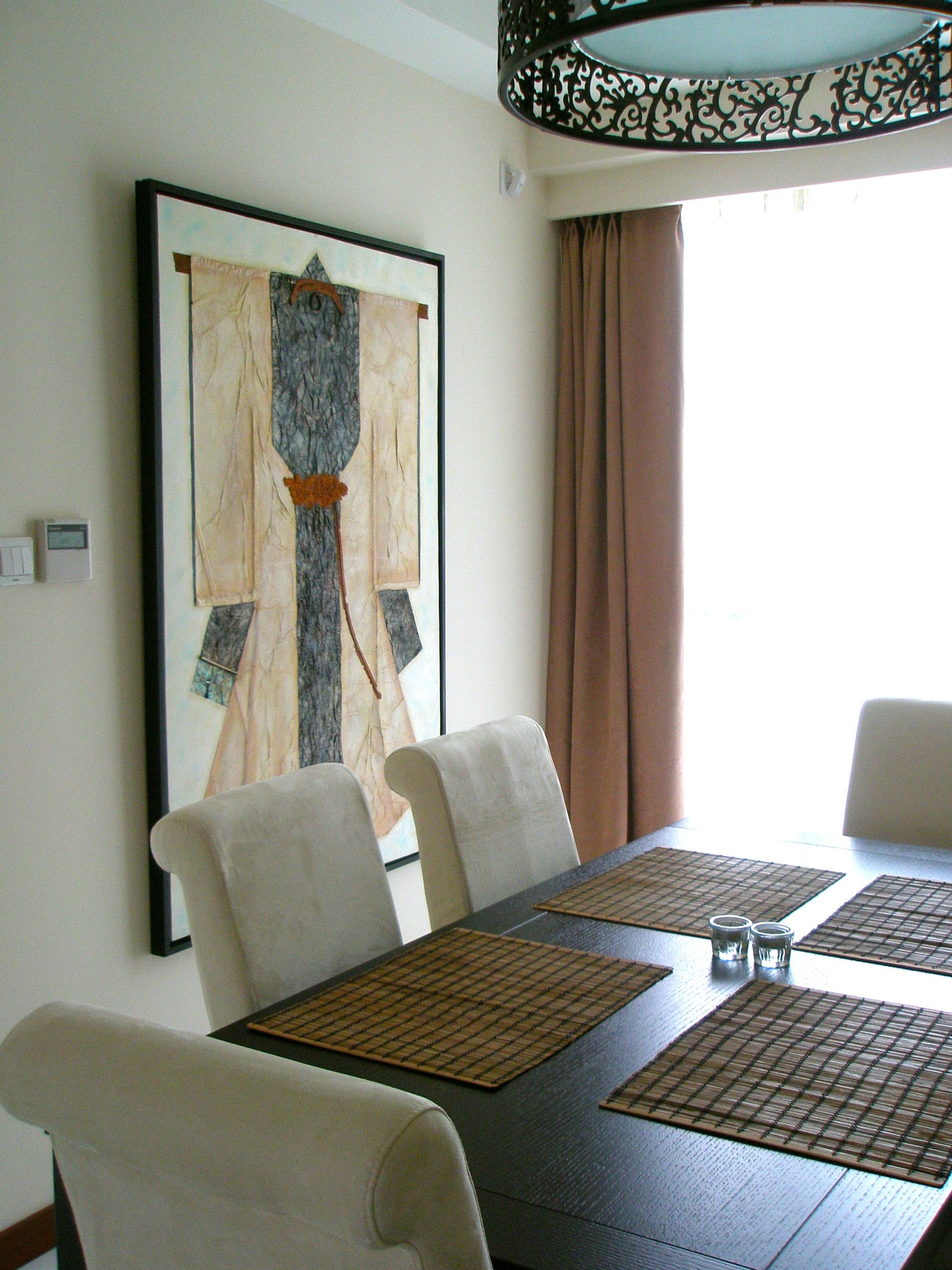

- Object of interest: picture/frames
[127,176,456,960]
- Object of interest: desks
[169,807,952,1270]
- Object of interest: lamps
[488,0,951,153]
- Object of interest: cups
[708,914,753,960]
[750,921,794,968]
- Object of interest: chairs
[383,713,584,935]
[2,997,495,1269]
[843,695,949,853]
[144,763,407,1038]
